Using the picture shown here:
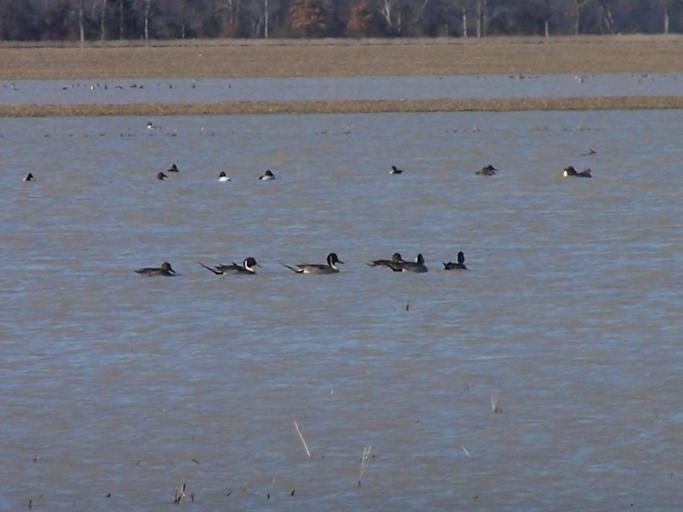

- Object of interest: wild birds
[20,170,35,182]
[145,122,162,131]
[258,169,275,181]
[560,164,592,179]
[280,253,345,276]
[129,262,176,277]
[217,170,231,182]
[155,163,181,180]
[441,252,468,271]
[388,163,402,175]
[475,163,497,176]
[197,257,263,277]
[364,253,428,274]
